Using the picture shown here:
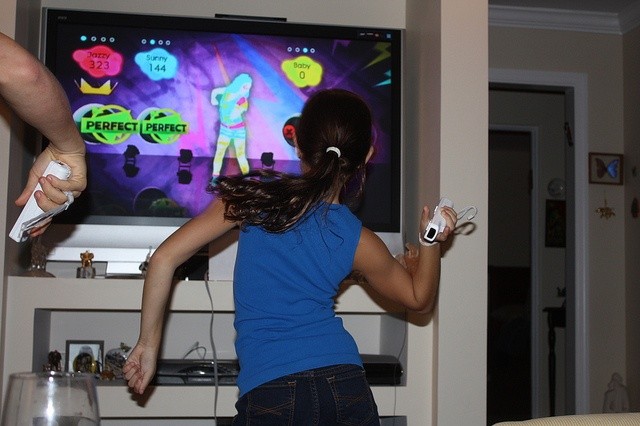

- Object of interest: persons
[206,73,252,186]
[120,89,459,425]
[1,33,88,244]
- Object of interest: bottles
[0,369,101,426]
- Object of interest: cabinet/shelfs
[3,266,430,426]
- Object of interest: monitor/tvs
[36,0,405,281]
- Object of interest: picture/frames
[65,340,104,374]
[590,152,630,184]
